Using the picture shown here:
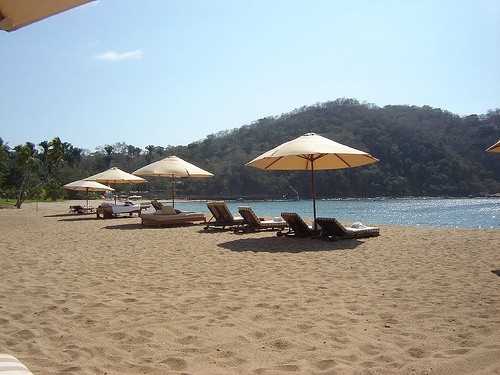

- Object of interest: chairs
[69,204,94,214]
[229,205,288,235]
[313,217,379,241]
[277,212,314,238]
[200,202,264,233]
[95,204,141,220]
[141,199,206,228]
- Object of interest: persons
[99,204,111,208]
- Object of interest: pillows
[160,206,176,215]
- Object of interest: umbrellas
[245,130,379,237]
[63,180,115,212]
[83,167,147,205]
[132,153,214,209]
